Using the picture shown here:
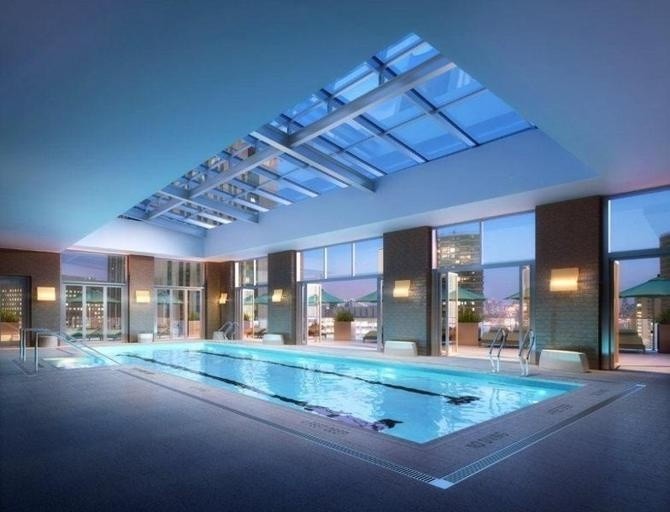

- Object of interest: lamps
[135,290,150,304]
[271,288,283,303]
[36,284,57,302]
[547,267,581,293]
[217,292,229,306]
[392,279,412,298]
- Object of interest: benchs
[535,347,590,374]
[261,333,285,347]
[383,339,420,359]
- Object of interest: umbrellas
[503,287,530,300]
[308,288,346,305]
[354,285,383,302]
[619,274,670,350]
[158,292,184,323]
[244,295,253,305]
[254,293,268,304]
[66,288,121,320]
[442,284,488,301]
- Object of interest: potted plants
[456,306,484,348]
[329,305,357,342]
[652,307,670,354]
[137,332,153,345]
[39,335,58,348]
